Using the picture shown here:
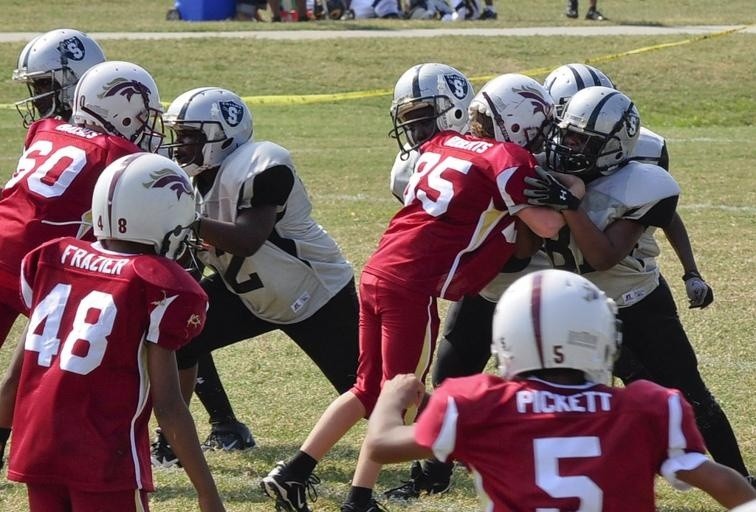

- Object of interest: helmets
[543,62,617,117]
[545,85,641,177]
[11,28,106,129]
[156,85,255,177]
[386,62,476,161]
[467,72,557,155]
[490,268,625,387]
[90,151,197,260]
[71,59,167,154]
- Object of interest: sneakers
[260,460,322,512]
[150,427,183,468]
[199,421,256,454]
[585,8,604,21]
[383,461,455,500]
[340,498,390,512]
[478,8,497,20]
[566,6,578,18]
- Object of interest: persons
[150,86,362,471]
[566,0,609,21]
[13,24,261,452]
[6,150,232,510]
[259,52,756,512]
[0,57,162,469]
[236,0,499,23]
[259,62,587,510]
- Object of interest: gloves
[682,271,714,309]
[522,164,580,214]
